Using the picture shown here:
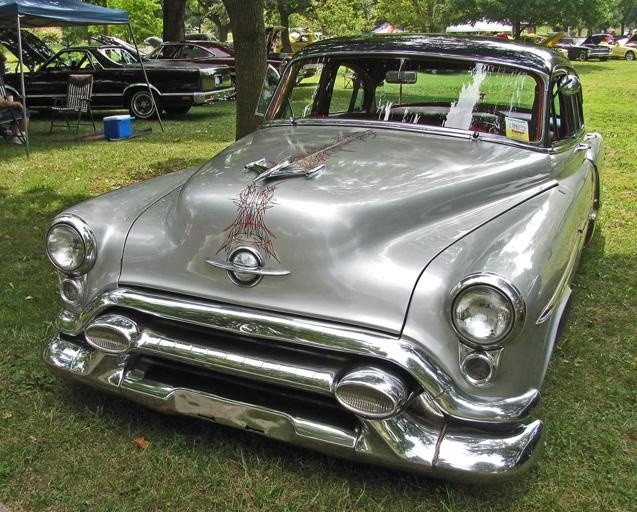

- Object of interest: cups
[6,94,13,102]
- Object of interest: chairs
[342,67,355,88]
[0,84,27,145]
[49,72,99,134]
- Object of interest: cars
[5,47,234,125]
[35,33,606,489]
[612,29,637,62]
[539,33,615,62]
[90,34,288,99]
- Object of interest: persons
[0,73,31,145]
[272,35,282,54]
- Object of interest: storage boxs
[103,114,135,139]
